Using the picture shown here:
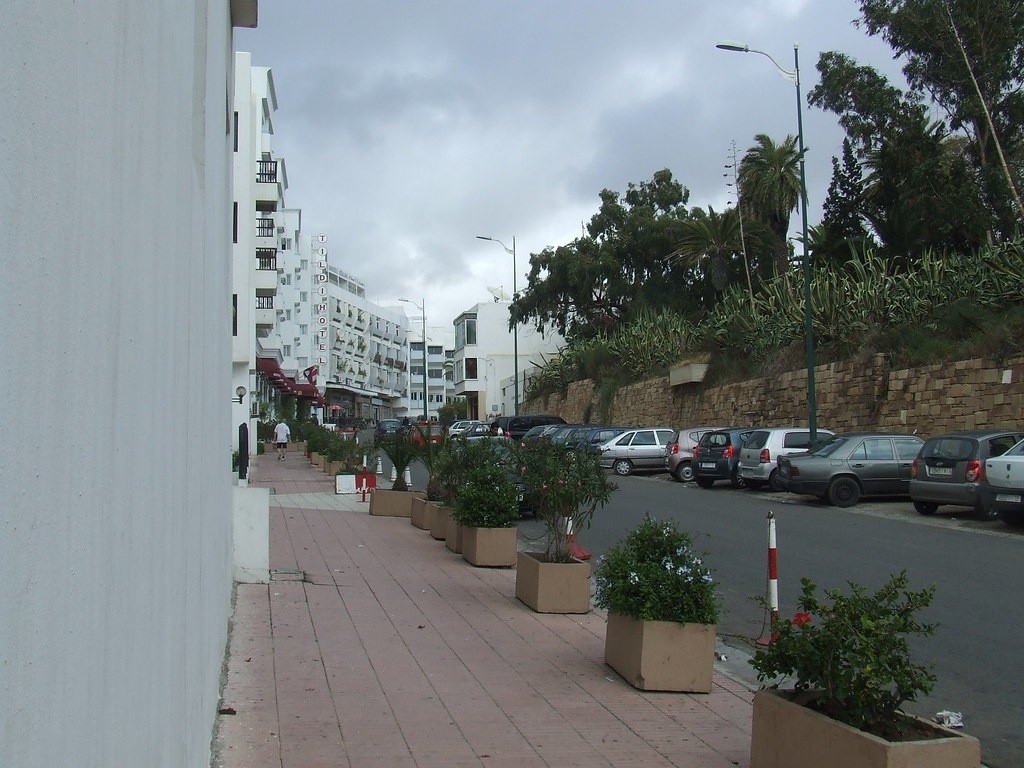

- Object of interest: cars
[664,426,735,482]
[518,424,629,465]
[592,428,678,476]
[445,445,545,522]
[907,431,1024,521]
[982,438,1024,526]
[376,419,403,438]
[776,431,946,507]
[690,427,760,490]
[410,419,514,460]
[738,428,871,493]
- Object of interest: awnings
[256,358,325,407]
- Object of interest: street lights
[475,234,519,416]
[398,296,429,421]
[714,39,818,448]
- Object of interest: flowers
[750,571,940,731]
[599,515,718,621]
[445,437,523,525]
[519,433,621,562]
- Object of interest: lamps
[232,386,246,404]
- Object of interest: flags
[303,366,316,385]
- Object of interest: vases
[515,550,592,614]
[607,608,722,694]
[750,686,980,768]
[460,524,520,569]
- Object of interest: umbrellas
[329,405,341,418]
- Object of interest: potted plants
[301,412,471,614]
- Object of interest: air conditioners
[251,401,259,415]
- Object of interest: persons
[487,422,500,436]
[403,416,409,426]
[275,418,292,461]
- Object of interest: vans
[493,415,569,445]
